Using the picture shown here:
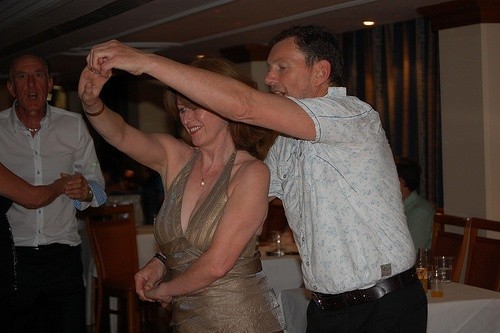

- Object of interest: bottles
[416,247,435,293]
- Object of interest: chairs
[85,204,160,333]
[428,213,470,283]
[464,216,500,292]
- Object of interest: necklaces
[27,128,38,131]
[200,168,207,186]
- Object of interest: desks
[86,194,500,333]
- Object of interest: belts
[311,266,419,310]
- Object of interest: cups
[434,256,455,283]
[268,233,286,256]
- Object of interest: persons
[78,56,286,333]
[0,55,107,333]
[86,25,429,333]
[396,158,434,259]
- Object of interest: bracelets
[86,105,105,116]
[89,188,93,200]
[154,253,166,264]
